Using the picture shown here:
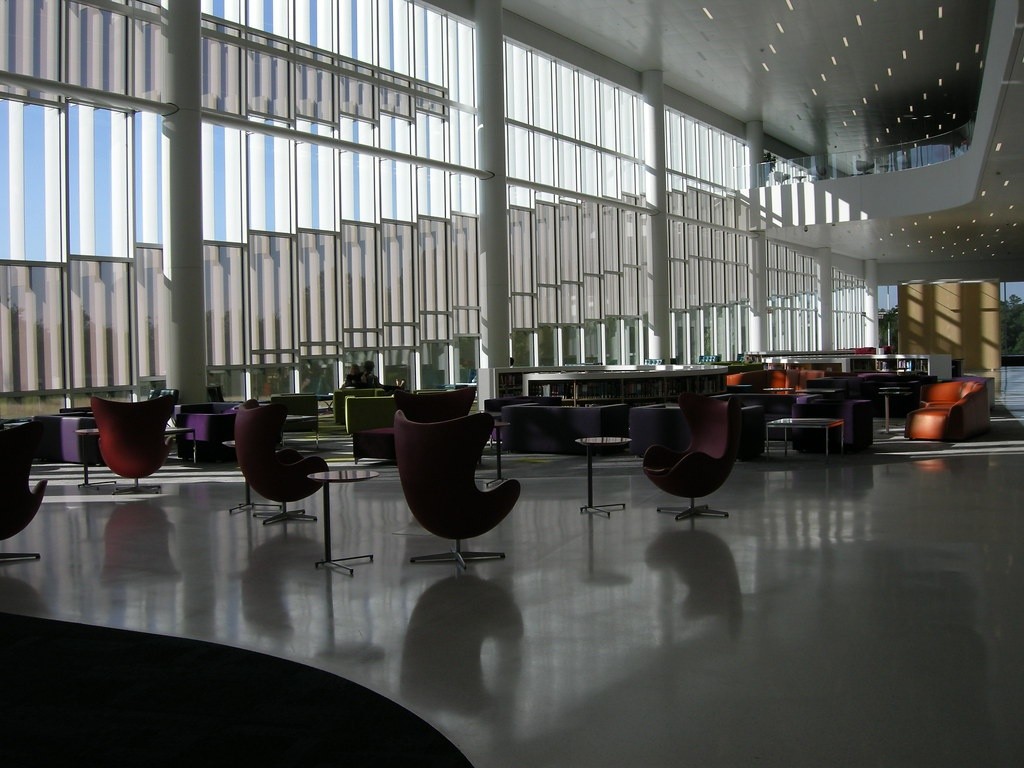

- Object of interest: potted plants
[759,151,776,186]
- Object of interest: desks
[793,176,807,182]
[878,387,913,435]
[221,441,282,515]
[480,412,511,488]
[766,418,845,462]
[307,469,380,574]
[75,427,117,488]
[796,389,844,393]
[166,427,196,464]
[880,165,893,172]
[763,387,793,393]
[575,437,633,517]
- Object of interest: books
[877,360,928,371]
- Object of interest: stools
[353,428,396,464]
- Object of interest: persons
[345,364,363,387]
[361,361,379,384]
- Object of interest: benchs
[33,411,100,467]
[858,372,937,417]
[904,379,991,441]
[484,396,562,441]
[708,393,823,441]
[501,403,629,453]
[174,402,269,462]
[630,403,765,461]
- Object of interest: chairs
[941,377,996,411]
[807,166,825,181]
[91,394,174,496]
[271,393,318,432]
[856,160,875,175]
[334,384,476,435]
[393,410,520,572]
[643,392,742,520]
[792,399,874,454]
[806,376,863,399]
[235,399,329,525]
[774,171,790,184]
[0,420,48,560]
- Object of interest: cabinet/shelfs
[478,365,729,413]
[761,354,952,380]
